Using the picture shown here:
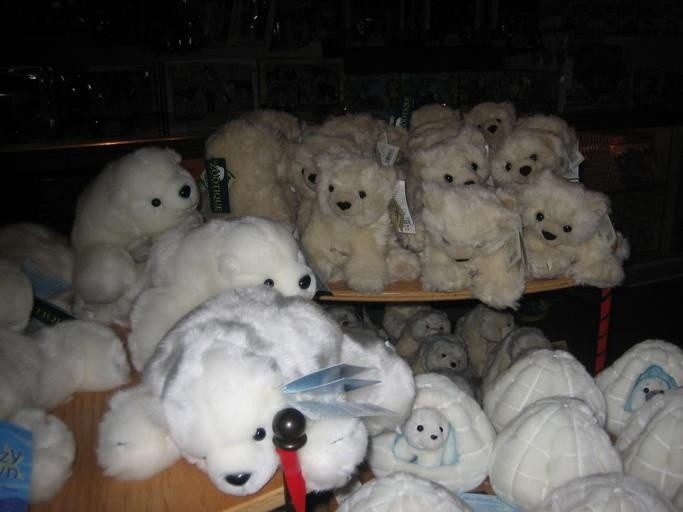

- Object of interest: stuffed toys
[1,99,683,512]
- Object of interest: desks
[315,269,612,379]
[15,322,289,511]
